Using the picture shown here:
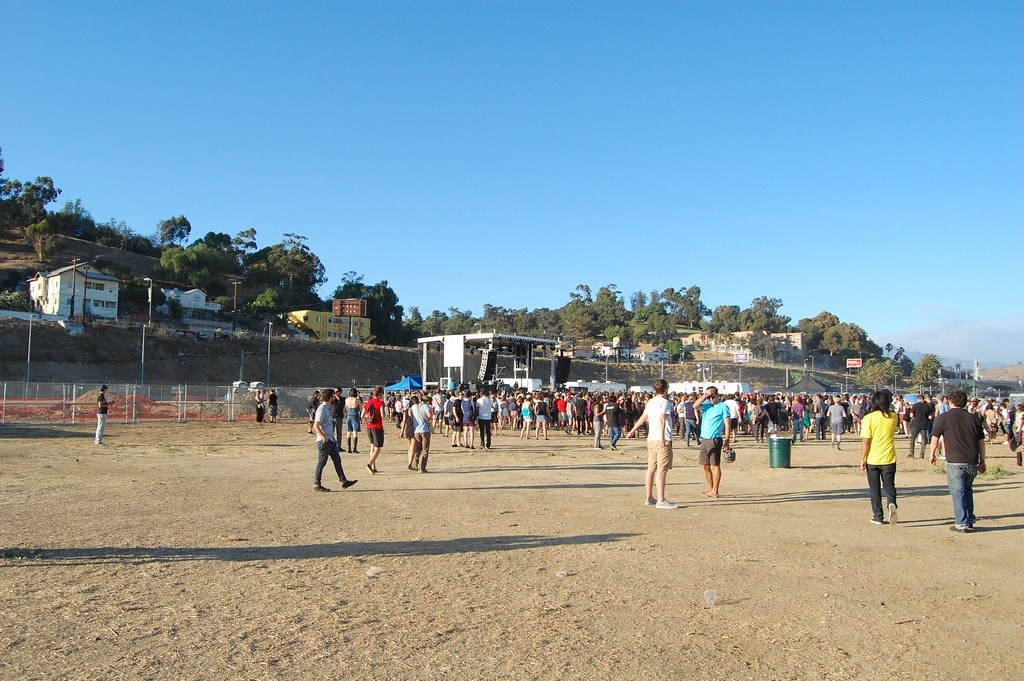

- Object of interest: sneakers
[610,443,617,451]
[645,498,657,505]
[656,500,678,509]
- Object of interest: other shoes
[451,444,457,447]
[314,486,330,492]
[341,479,358,489]
[831,441,841,450]
[871,519,882,524]
[949,524,974,533]
[466,445,470,448]
[889,503,898,525]
[1002,440,1008,444]
[365,464,379,474]
[471,446,476,449]
[457,443,464,446]
[339,447,345,451]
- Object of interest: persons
[255,389,278,423]
[313,389,358,492]
[840,392,1024,466]
[591,390,847,450]
[361,386,386,475]
[860,391,898,525]
[929,391,986,533]
[693,386,731,498]
[306,387,364,453]
[452,389,495,451]
[627,379,679,509]
[95,384,113,445]
[367,378,595,441]
[400,396,432,474]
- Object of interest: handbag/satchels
[768,420,775,433]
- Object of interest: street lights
[809,356,813,370]
[144,278,153,327]
[141,325,147,386]
[268,322,274,385]
[804,359,808,369]
[26,314,36,401]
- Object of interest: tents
[777,374,839,393]
[384,375,423,417]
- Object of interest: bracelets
[980,459,985,463]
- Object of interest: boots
[347,437,352,453]
[352,437,359,454]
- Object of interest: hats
[99,384,108,389]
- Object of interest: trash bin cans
[768,437,792,468]
